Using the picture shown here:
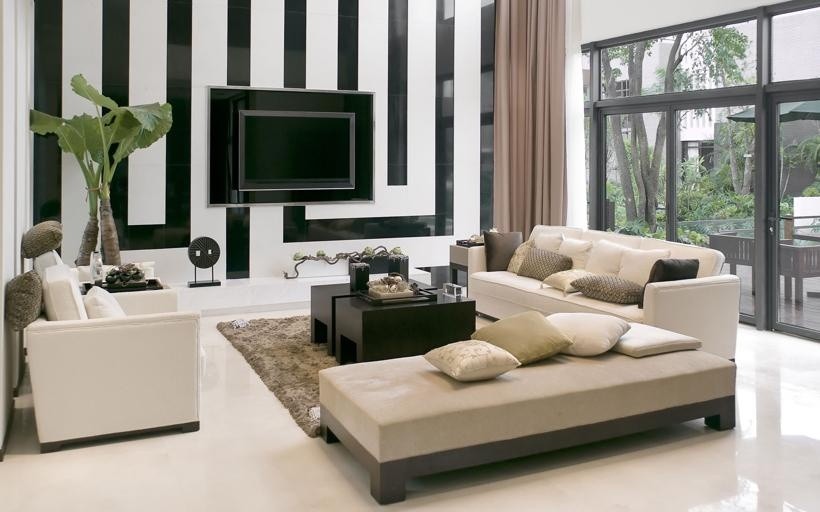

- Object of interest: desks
[449,243,486,298]
[80,274,164,298]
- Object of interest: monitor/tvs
[238,110,356,192]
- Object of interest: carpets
[215,313,348,441]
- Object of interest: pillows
[79,278,128,325]
[605,319,707,364]
[483,226,701,310]
[470,306,575,371]
[544,308,634,358]
[19,216,66,260]
[3,270,46,334]
[424,338,525,387]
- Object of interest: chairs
[31,248,157,285]
[21,262,201,456]
[706,228,819,305]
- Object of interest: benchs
[316,346,739,506]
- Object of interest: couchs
[466,222,742,361]
